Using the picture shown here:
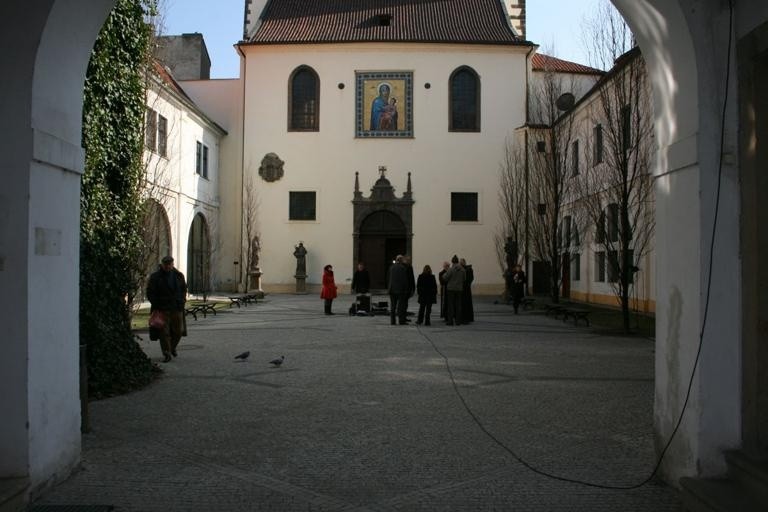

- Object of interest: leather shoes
[171,350,178,358]
[163,355,172,362]
[402,319,421,325]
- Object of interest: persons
[293,242,307,275]
[350,262,370,294]
[320,264,337,315]
[146,256,187,363]
[387,253,475,327]
[507,264,524,315]
[371,86,398,130]
[250,234,261,270]
[504,236,518,269]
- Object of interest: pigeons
[269,355,285,367]
[234,351,251,361]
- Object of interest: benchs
[544,303,589,327]
[520,300,537,311]
[184,302,217,320]
[229,293,258,308]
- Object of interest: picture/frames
[355,69,414,138]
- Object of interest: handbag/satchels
[149,311,165,341]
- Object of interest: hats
[162,256,173,262]
